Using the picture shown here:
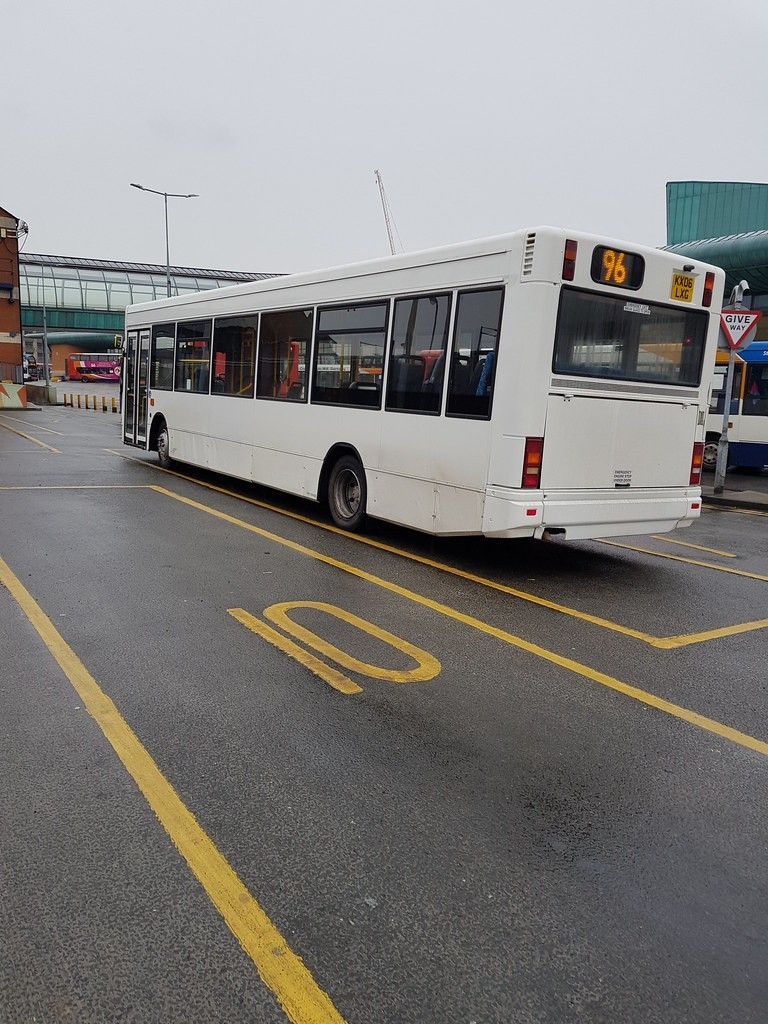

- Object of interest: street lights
[131,183,199,298]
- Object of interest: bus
[67,353,122,383]
[117,227,726,543]
